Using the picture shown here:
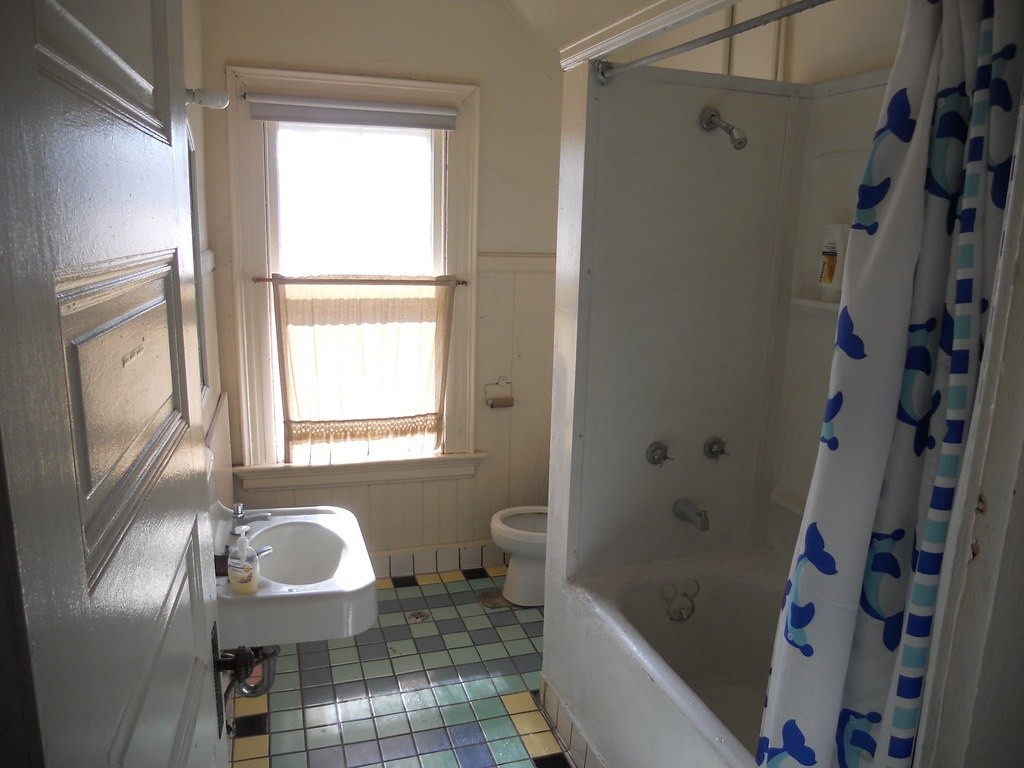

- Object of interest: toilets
[490,506,550,606]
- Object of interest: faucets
[231,503,271,535]
[673,500,710,531]
[247,546,273,563]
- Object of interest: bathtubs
[540,557,794,768]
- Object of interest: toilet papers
[484,377,512,401]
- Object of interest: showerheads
[714,121,747,149]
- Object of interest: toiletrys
[819,240,837,282]
[227,526,259,595]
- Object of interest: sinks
[215,501,379,650]
